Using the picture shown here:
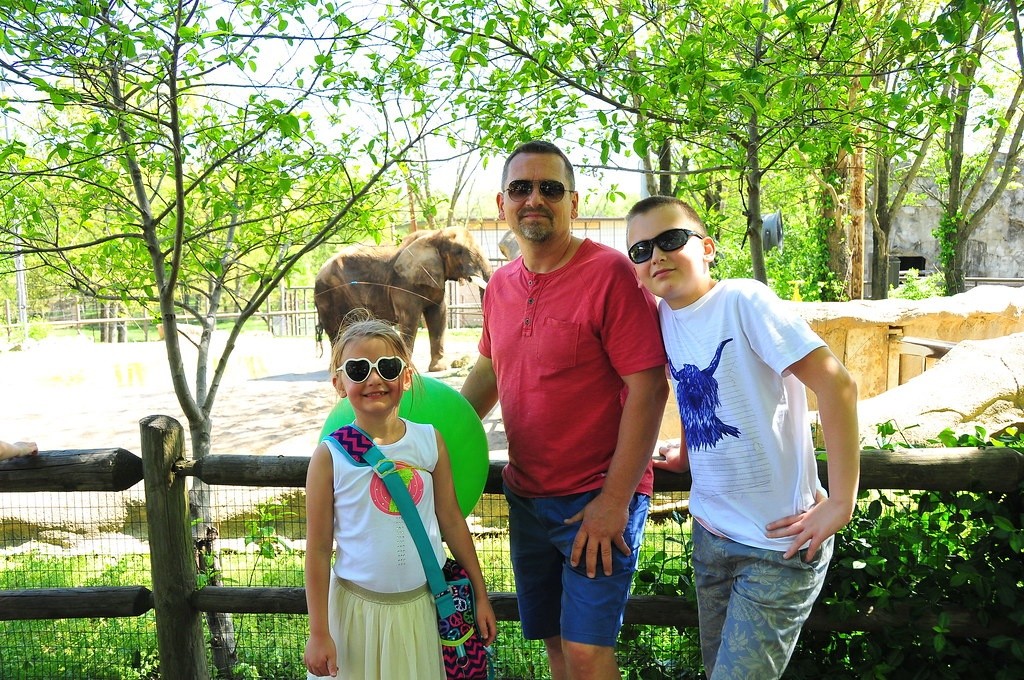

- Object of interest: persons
[459,140,670,680]
[625,196,860,680]
[303,314,497,680]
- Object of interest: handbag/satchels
[429,557,487,680]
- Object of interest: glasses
[336,356,406,383]
[503,180,574,203]
[628,229,703,264]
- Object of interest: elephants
[312,225,494,373]
